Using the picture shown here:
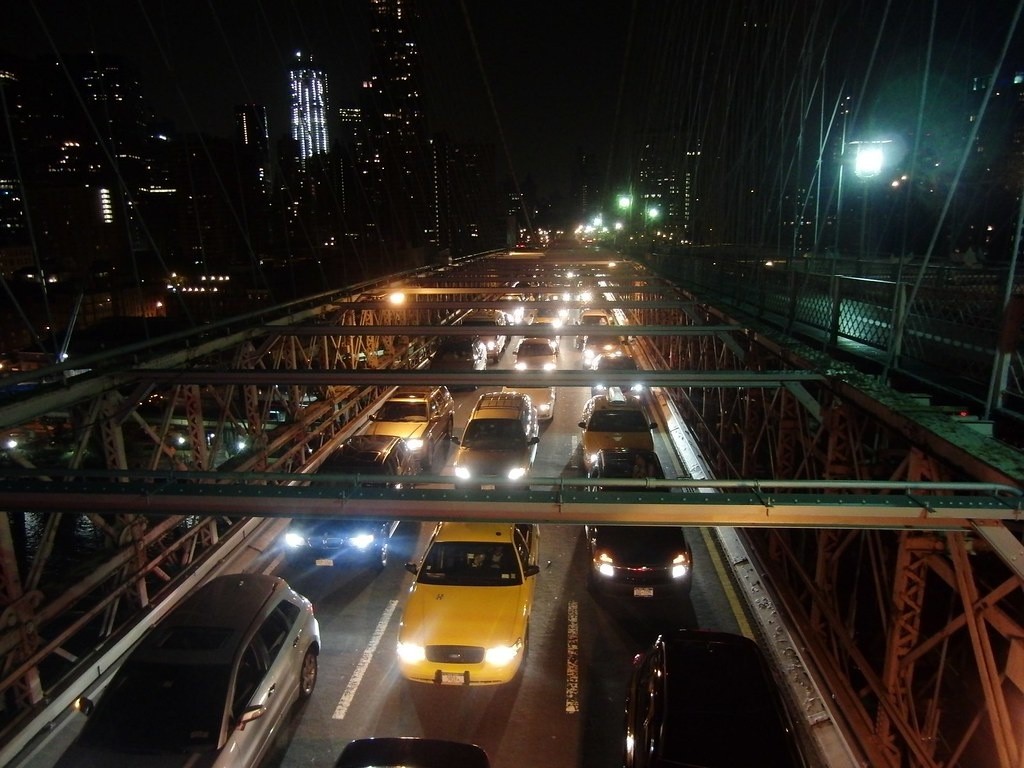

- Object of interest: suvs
[450,392,540,490]
[365,385,455,467]
[575,310,615,347]
[428,338,487,390]
[457,309,511,362]
[626,629,795,768]
[499,296,524,320]
[75,569,321,768]
[585,448,693,599]
[286,435,417,569]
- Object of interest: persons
[484,544,509,571]
[634,454,655,476]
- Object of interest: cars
[398,521,539,685]
[530,309,561,325]
[578,387,658,470]
[512,338,559,370]
[588,352,644,398]
[502,385,556,421]
[545,292,570,301]
[583,335,622,367]
